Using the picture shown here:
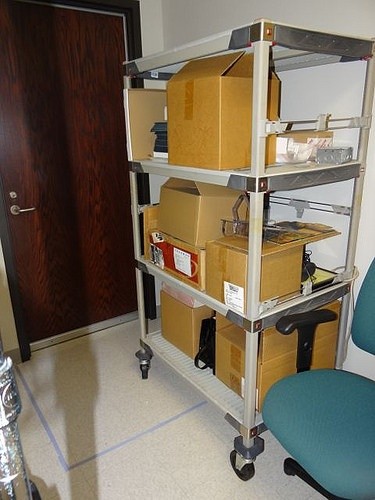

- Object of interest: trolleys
[120,19,375,483]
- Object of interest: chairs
[261,257,375,500]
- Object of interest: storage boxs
[155,178,248,247]
[203,241,305,313]
[214,299,343,409]
[318,145,355,165]
[159,280,215,360]
[275,129,333,162]
[148,227,208,291]
[165,52,281,171]
